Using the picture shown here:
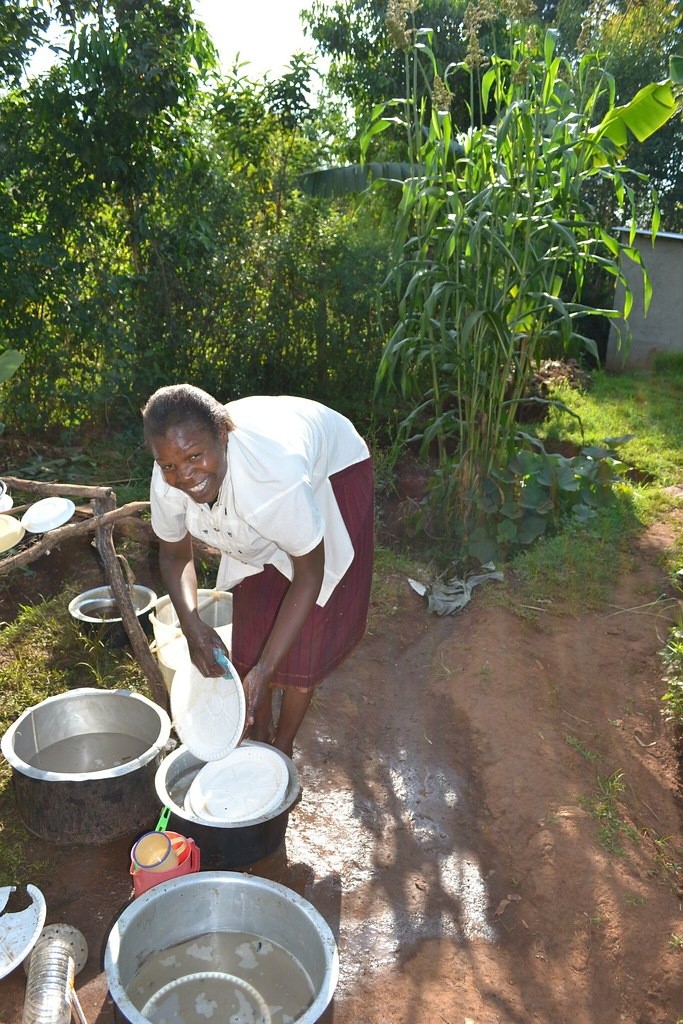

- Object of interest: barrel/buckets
[151,588,233,693]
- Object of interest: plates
[141,972,271,1024]
[170,652,246,761]
[0,479,76,554]
[183,747,289,821]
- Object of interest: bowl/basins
[74,598,140,618]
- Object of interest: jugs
[129,831,200,899]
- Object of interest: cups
[133,831,187,872]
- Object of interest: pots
[68,583,158,648]
[1,688,171,844]
[103,871,339,1024]
[155,740,303,866]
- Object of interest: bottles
[22,939,76,1024]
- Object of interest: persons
[142,383,375,758]
